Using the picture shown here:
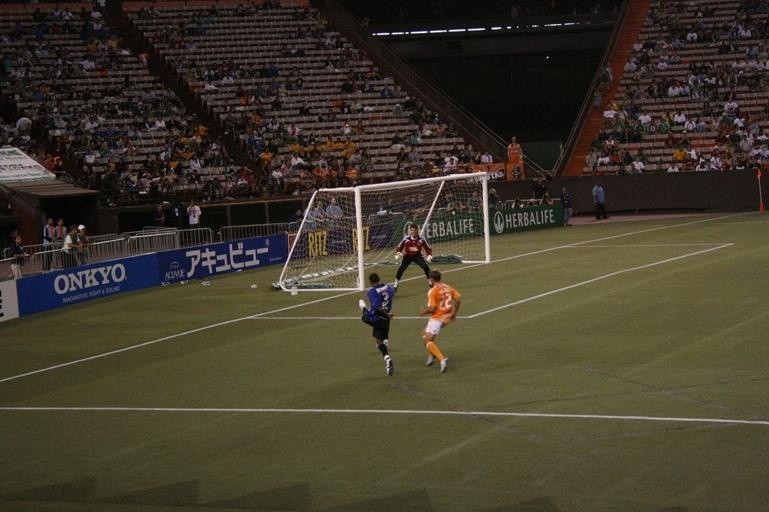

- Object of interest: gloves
[395,252,402,259]
[427,254,432,262]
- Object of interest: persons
[0,0,768,281]
[358,272,398,376]
[418,270,462,372]
[394,224,435,288]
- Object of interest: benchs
[580,1,768,172]
[1,0,505,208]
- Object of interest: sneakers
[426,355,435,365]
[359,299,366,309]
[385,355,393,376]
[441,357,448,373]
